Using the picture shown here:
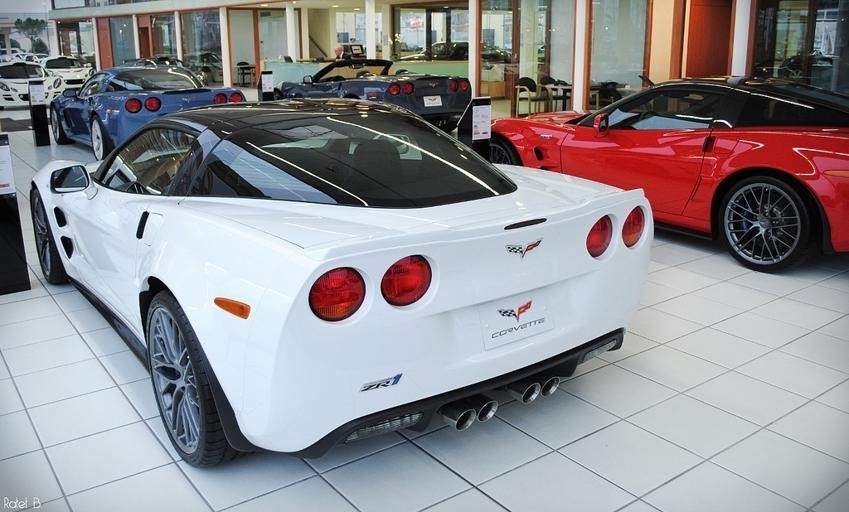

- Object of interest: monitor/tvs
[283,55,292,62]
[350,44,365,57]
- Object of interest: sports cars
[25,94,659,473]
[468,77,849,276]
[0,45,97,112]
[270,55,474,135]
[47,64,250,163]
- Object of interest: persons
[335,46,351,59]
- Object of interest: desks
[536,83,599,113]
[233,65,255,88]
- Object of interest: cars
[386,39,507,81]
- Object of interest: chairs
[514,77,568,118]
[348,140,406,185]
[237,62,251,87]
[598,82,622,107]
